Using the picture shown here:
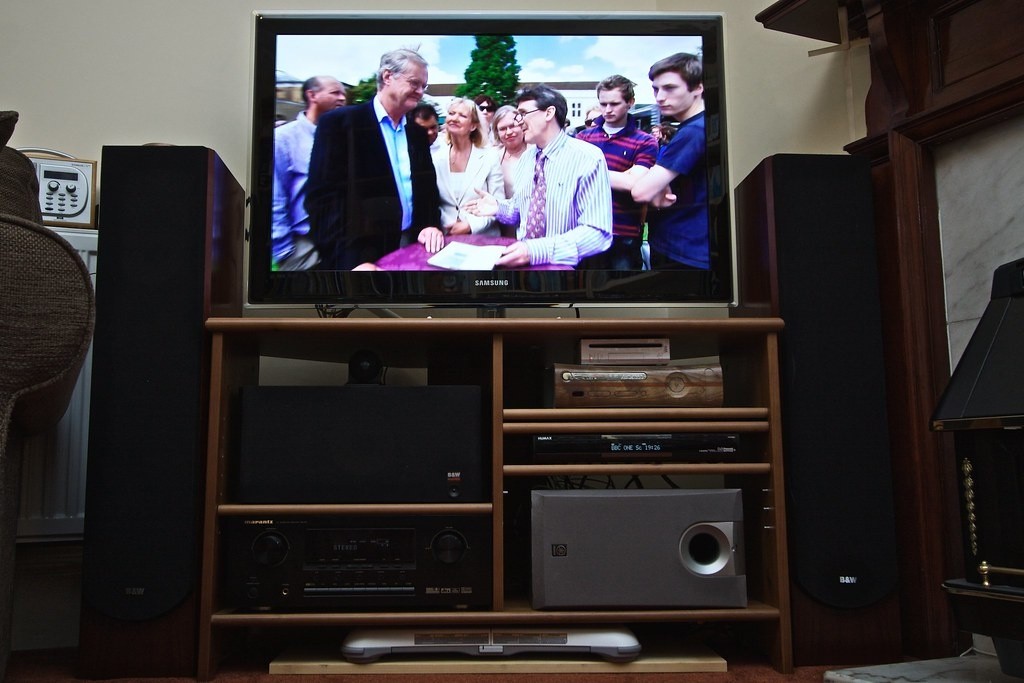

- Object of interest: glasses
[585,118,596,126]
[480,105,494,112]
[395,70,428,91]
[514,109,545,123]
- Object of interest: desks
[755,0,1024,683]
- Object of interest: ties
[525,151,547,240]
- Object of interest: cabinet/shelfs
[14,220,99,653]
[196,315,794,683]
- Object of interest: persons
[271,46,710,271]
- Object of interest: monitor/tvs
[242,15,738,323]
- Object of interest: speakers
[733,154,911,670]
[73,145,247,679]
[528,489,748,612]
[238,382,493,505]
